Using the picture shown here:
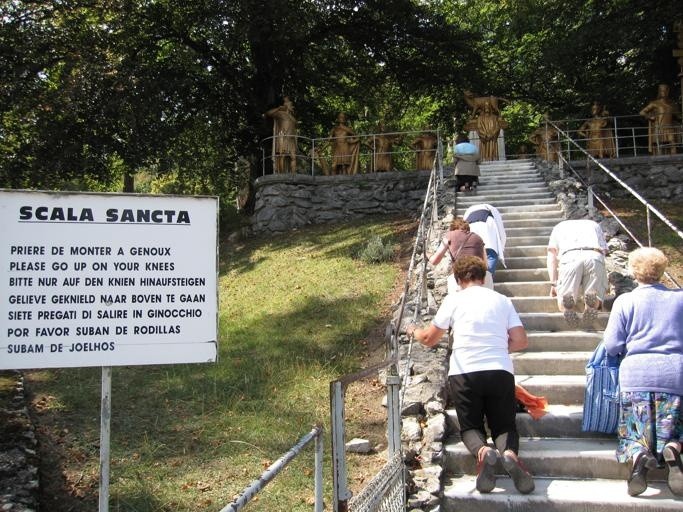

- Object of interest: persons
[476,102,500,163]
[599,109,616,158]
[599,245,682,497]
[363,124,406,173]
[410,124,436,169]
[462,203,506,283]
[403,251,539,495]
[264,95,303,174]
[546,219,608,331]
[452,134,480,192]
[526,111,566,162]
[575,102,606,160]
[316,112,360,177]
[515,144,528,158]
[638,82,683,156]
[421,218,494,351]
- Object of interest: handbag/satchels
[581,362,619,434]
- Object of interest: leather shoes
[662,446,683,497]
[628,452,658,496]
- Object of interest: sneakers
[563,293,579,328]
[582,291,599,326]
[465,181,477,192]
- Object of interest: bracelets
[549,283,555,287]
[410,327,417,339]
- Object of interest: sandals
[500,450,535,494]
[476,446,498,492]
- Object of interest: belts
[562,247,604,255]
